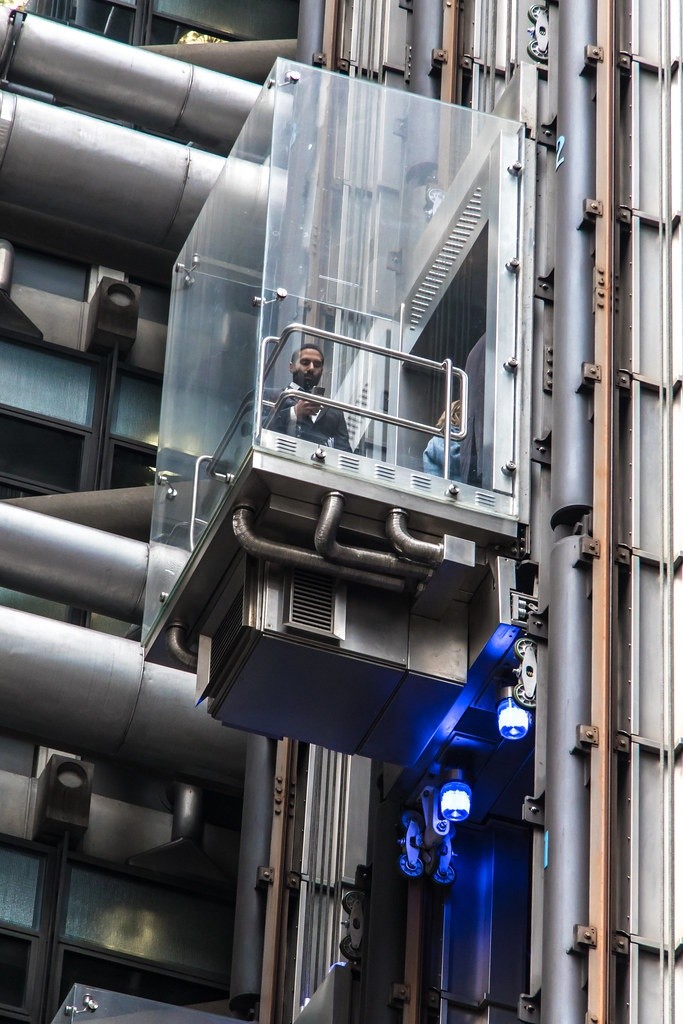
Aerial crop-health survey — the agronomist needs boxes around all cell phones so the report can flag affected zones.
[312,386,325,397]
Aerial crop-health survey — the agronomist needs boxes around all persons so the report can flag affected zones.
[423,400,462,483]
[262,343,353,454]
